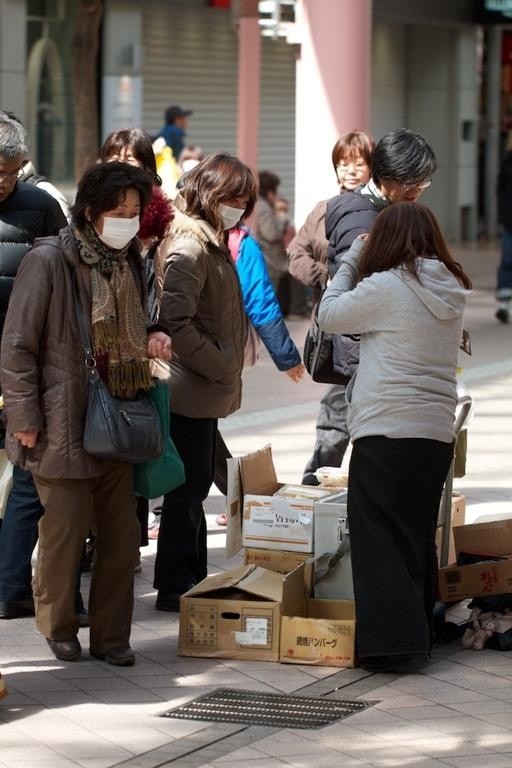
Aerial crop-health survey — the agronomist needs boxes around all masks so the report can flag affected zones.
[91,213,140,249]
[213,203,245,230]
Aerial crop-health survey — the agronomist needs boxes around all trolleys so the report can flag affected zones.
[432,395,512,651]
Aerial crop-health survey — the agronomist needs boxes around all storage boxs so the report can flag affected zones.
[223,444,315,554]
[242,548,315,600]
[314,489,356,600]
[433,444,510,654]
[180,563,281,664]
[279,561,358,666]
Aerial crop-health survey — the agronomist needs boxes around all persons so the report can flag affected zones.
[101,107,304,617]
[311,201,472,677]
[286,128,438,487]
[1,110,73,617]
[495,121,511,325]
[3,160,175,668]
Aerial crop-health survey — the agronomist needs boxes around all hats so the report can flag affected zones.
[165,106,193,120]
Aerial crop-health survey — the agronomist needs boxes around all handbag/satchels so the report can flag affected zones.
[83,370,164,465]
[129,378,187,500]
[303,299,347,384]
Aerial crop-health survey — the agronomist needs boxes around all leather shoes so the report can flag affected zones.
[89,641,135,666]
[156,580,195,611]
[78,609,90,627]
[47,635,82,661]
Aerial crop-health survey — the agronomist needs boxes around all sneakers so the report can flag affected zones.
[0,598,34,618]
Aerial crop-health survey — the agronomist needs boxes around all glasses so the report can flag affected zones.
[398,177,432,191]
[0,167,25,183]
[336,161,367,172]
